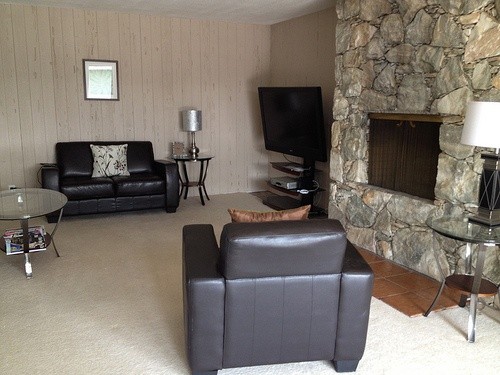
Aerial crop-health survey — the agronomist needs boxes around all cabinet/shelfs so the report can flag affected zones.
[265,161,324,217]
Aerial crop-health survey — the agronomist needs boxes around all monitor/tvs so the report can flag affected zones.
[257,86,328,171]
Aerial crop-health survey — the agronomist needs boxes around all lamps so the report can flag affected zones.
[182,109,203,155]
[461,101,500,228]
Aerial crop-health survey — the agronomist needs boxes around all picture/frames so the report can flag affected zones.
[83,59,120,101]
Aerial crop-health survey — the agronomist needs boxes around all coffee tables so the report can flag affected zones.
[0,185,66,279]
[172,153,214,206]
[426,203,500,344]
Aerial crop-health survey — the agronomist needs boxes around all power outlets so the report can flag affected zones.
[8,185,16,192]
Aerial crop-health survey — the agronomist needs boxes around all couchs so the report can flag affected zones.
[37,141,180,224]
[182,204,375,375]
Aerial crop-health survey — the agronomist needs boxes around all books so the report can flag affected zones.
[4,226,47,255]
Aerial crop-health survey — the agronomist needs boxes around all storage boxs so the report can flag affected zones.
[3,225,47,255]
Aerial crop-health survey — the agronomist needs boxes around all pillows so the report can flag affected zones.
[226,204,311,224]
[90,144,131,178]
